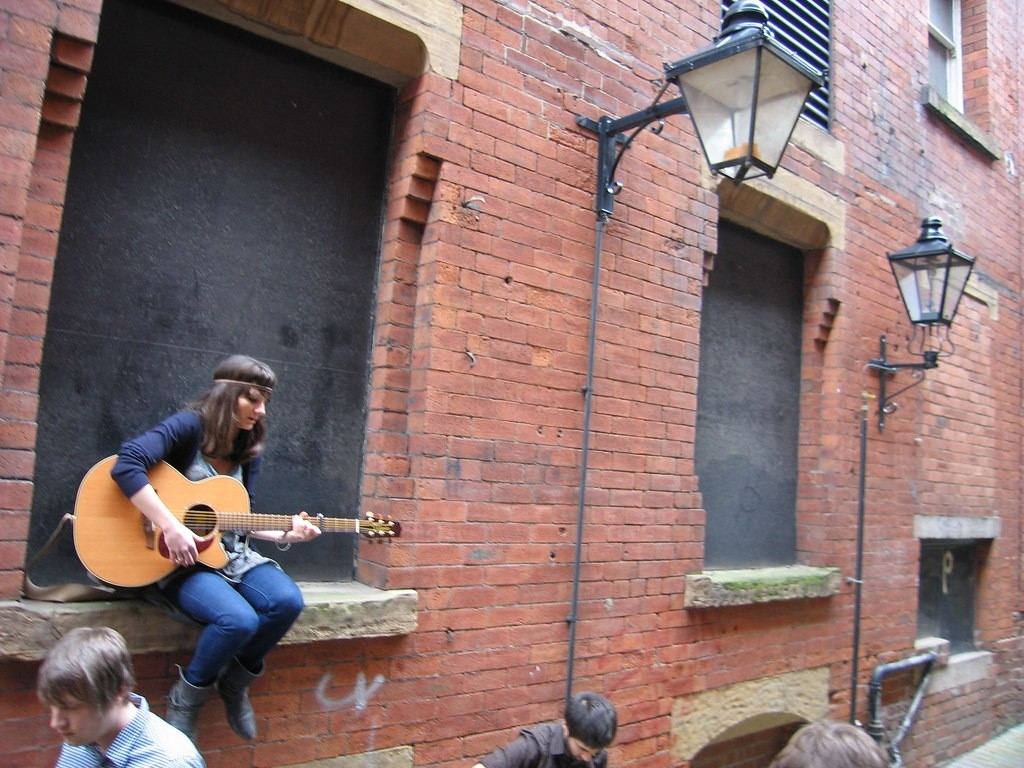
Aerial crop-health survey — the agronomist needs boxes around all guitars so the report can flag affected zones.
[72,452,402,595]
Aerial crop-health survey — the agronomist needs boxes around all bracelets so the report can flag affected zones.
[274,532,292,552]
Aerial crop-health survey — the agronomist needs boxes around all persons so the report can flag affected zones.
[110,354,323,745]
[471,691,618,768]
[34,625,208,768]
[767,718,894,768]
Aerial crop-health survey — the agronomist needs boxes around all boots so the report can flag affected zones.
[212,653,268,741]
[166,663,212,743]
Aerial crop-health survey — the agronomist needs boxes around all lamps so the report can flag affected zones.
[885,215,977,369]
[662,0,829,186]
